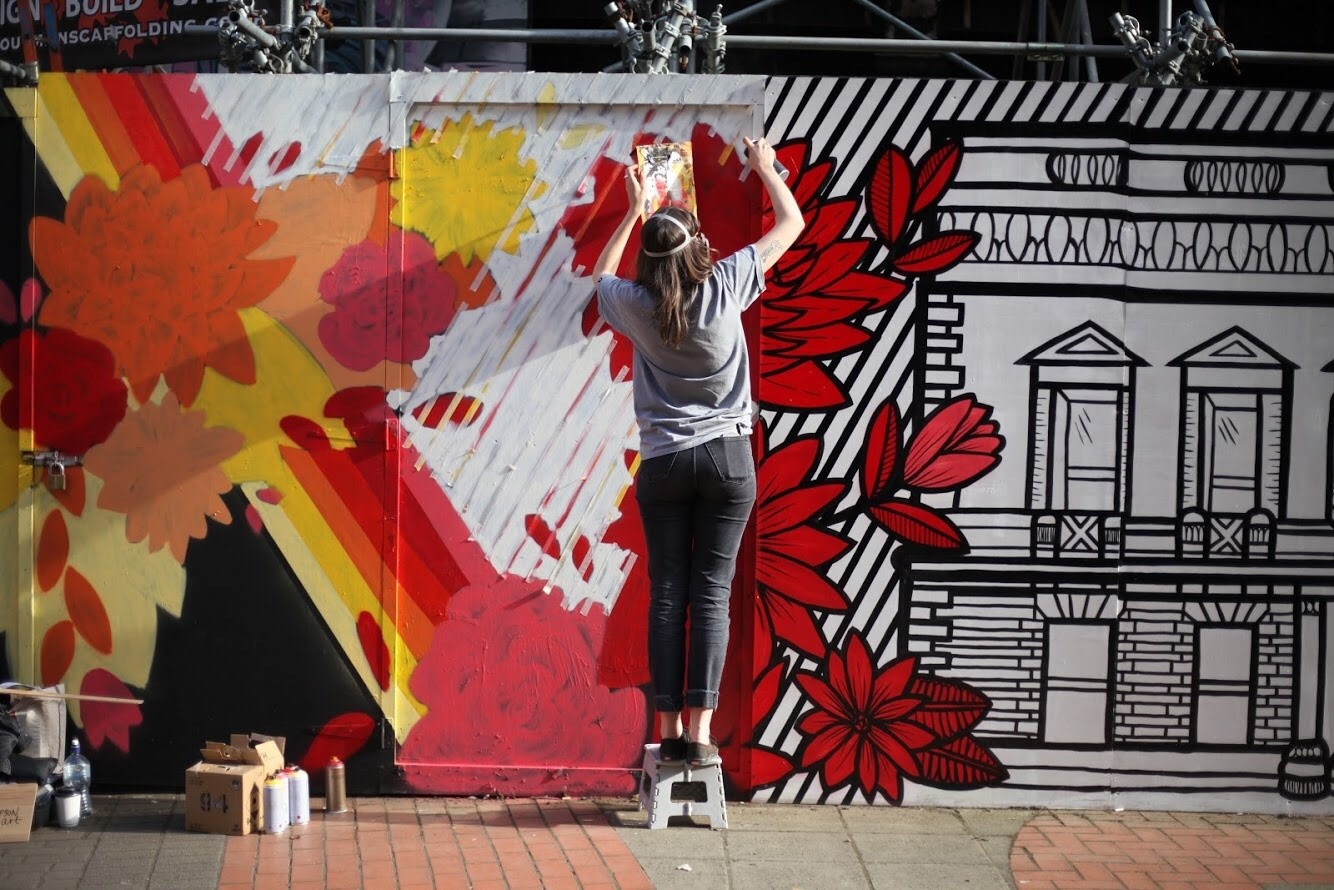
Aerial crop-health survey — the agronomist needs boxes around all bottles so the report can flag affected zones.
[325,756,347,814]
[743,140,790,182]
[274,761,310,826]
[63,739,93,819]
[264,776,286,835]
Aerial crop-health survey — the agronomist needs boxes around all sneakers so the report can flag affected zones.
[687,742,722,767]
[660,727,688,761]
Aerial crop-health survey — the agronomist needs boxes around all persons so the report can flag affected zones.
[593,136,805,765]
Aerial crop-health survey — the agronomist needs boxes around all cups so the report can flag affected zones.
[56,787,81,828]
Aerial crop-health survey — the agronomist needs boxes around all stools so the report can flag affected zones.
[637,743,729,831]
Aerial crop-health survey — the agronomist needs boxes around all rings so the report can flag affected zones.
[623,174,628,178]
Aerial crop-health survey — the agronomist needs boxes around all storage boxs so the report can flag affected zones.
[185,734,286,835]
[0,781,38,844]
